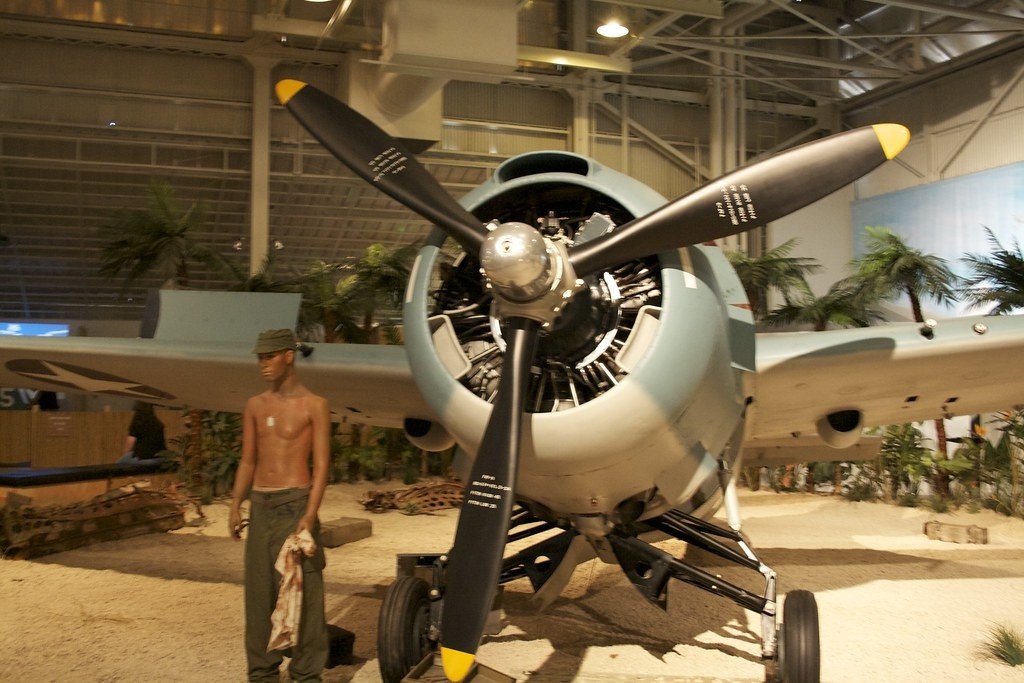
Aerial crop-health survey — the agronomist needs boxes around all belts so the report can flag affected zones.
[249,489,311,509]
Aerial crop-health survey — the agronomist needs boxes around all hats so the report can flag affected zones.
[252,328,297,355]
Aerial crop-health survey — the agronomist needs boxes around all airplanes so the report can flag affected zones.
[0,71,1024,683]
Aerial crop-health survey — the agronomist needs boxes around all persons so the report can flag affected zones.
[117,401,164,464]
[228,328,331,683]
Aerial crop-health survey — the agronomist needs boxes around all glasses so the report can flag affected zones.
[257,351,285,359]
